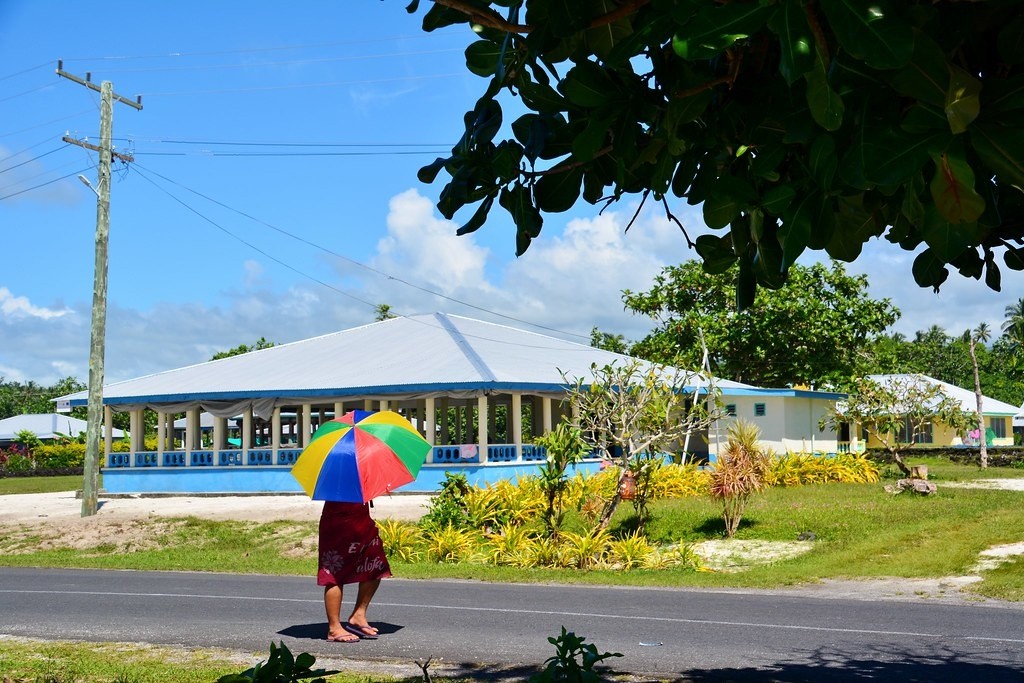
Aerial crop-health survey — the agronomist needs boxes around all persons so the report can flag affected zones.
[317,502,393,643]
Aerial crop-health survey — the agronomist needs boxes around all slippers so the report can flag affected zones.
[326,634,359,643]
[346,625,378,638]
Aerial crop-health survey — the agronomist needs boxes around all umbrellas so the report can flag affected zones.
[290,410,432,504]
